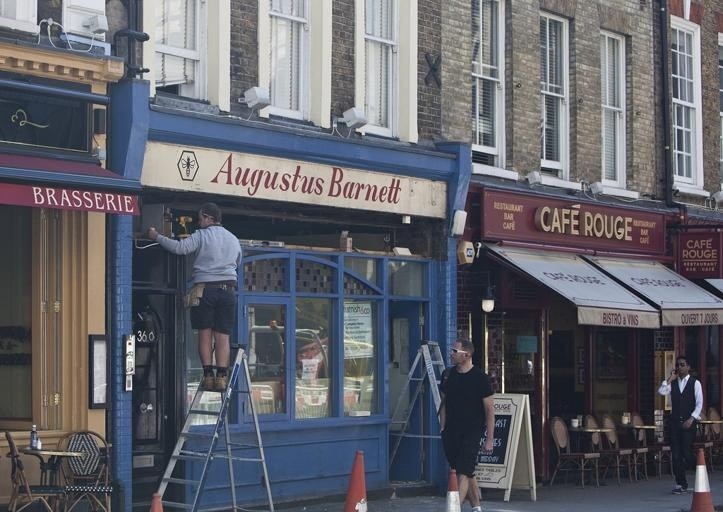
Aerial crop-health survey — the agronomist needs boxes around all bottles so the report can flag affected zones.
[29,425,41,451]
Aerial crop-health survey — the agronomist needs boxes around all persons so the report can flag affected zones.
[146,202,243,391]
[657,356,704,494]
[437,337,496,511]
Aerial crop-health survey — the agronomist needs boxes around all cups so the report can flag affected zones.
[621,416,628,424]
[570,419,578,429]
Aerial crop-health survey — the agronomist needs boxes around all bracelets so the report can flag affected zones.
[691,416,695,422]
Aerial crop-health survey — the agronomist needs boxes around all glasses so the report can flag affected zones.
[449,347,468,355]
[676,363,686,368]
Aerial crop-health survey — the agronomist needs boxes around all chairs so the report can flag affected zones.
[3,430,114,512]
[548,407,722,490]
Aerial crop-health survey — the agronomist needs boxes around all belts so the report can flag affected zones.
[205,284,226,288]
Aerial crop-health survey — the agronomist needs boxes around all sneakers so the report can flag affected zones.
[672,487,688,495]
[215,377,227,392]
[202,376,215,391]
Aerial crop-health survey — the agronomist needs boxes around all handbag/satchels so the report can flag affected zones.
[678,415,697,432]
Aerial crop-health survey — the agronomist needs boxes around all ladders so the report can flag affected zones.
[149,342,274,512]
[389,340,482,501]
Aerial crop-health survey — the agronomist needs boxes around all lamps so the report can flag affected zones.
[238,86,271,111]
[336,106,367,129]
[588,181,603,194]
[524,171,541,185]
[468,270,496,313]
[711,191,722,203]
[94,147,107,161]
[82,15,108,34]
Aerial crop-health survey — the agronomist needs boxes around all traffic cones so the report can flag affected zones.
[149,492,164,512]
[445,470,462,512]
[342,450,369,512]
[689,448,715,512]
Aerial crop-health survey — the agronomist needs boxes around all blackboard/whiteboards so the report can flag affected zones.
[473,393,525,490]
